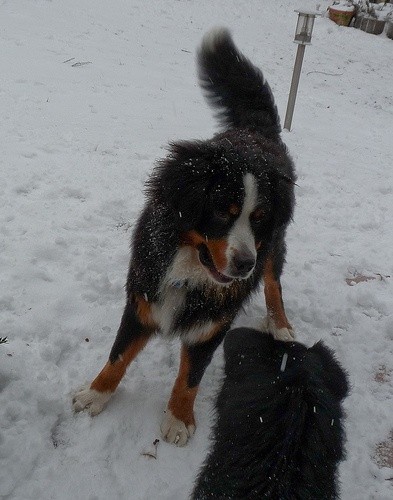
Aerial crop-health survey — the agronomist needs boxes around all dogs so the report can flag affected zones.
[187,326,352,500]
[72,27,298,448]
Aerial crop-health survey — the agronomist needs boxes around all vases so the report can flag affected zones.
[328,4,356,28]
[360,12,387,35]
